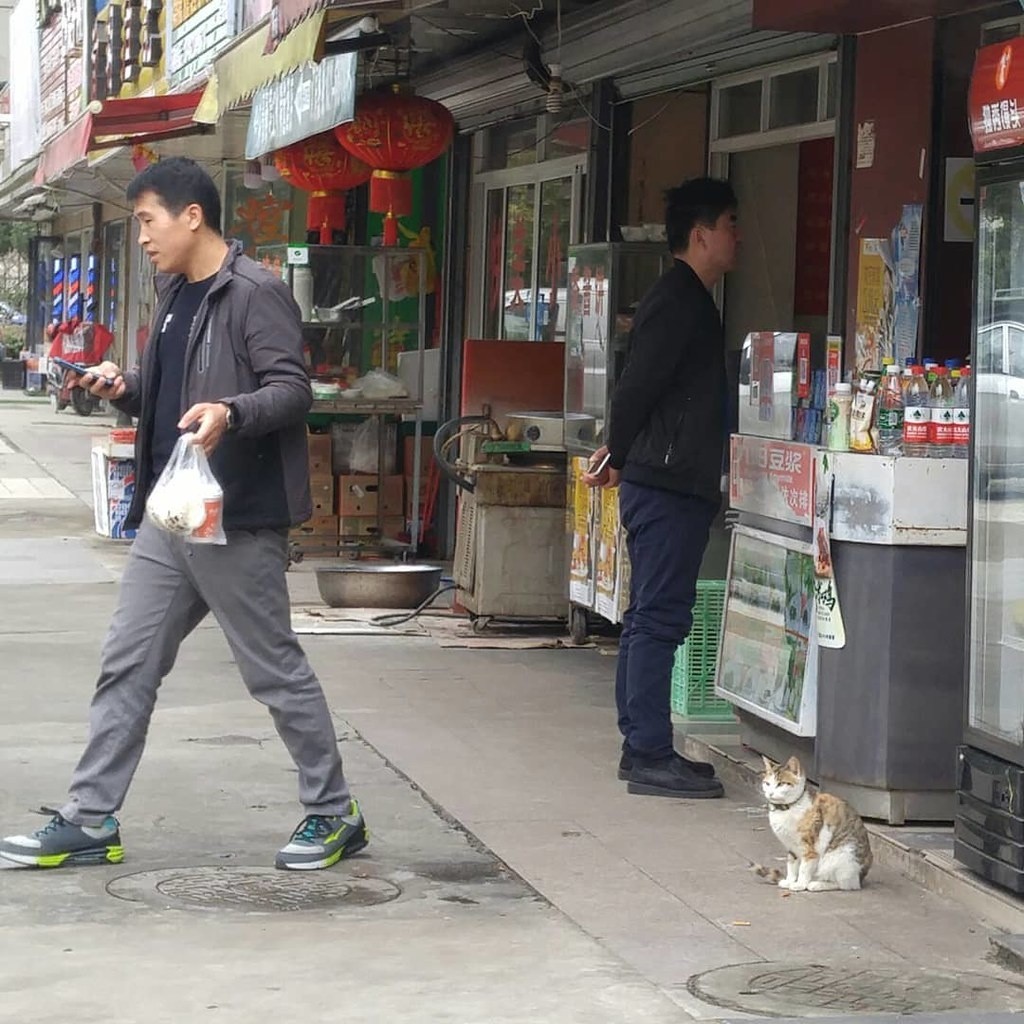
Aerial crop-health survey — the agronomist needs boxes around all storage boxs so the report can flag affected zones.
[288,424,435,558]
[671,580,735,722]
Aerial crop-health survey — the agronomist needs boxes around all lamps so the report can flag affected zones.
[261,152,280,181]
[244,160,262,189]
[12,193,65,222]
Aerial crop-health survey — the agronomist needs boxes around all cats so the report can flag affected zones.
[744,752,875,893]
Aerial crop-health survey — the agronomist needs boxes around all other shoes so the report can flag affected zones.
[618,751,716,780]
[628,760,725,798]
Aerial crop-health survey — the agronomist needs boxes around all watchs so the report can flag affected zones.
[226,405,234,429]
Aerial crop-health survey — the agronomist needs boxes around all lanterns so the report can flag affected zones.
[334,83,455,248]
[275,130,371,244]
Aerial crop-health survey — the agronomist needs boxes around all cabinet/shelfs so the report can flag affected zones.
[255,243,426,407]
[563,241,669,454]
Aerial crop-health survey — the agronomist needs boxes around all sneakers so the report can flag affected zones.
[0,806,123,869]
[275,798,372,870]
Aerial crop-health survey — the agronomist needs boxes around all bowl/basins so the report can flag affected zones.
[314,308,358,323]
[620,223,667,243]
[314,563,444,609]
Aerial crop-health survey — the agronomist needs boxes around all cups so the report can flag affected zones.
[184,495,222,543]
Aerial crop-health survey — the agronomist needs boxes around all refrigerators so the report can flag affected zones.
[950,43,1024,902]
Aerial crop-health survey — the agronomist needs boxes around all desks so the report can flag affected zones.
[286,398,421,562]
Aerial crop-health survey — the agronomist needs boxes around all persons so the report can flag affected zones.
[583,176,742,799]
[0,156,368,868]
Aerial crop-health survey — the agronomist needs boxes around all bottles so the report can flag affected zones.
[875,356,971,459]
[261,253,313,321]
[826,383,852,453]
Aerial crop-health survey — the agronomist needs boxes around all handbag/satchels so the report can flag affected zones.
[146,437,229,546]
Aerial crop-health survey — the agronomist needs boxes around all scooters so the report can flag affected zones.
[48,319,103,417]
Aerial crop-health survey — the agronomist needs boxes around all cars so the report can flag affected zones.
[737,330,800,398]
[978,320,1024,499]
[504,288,566,343]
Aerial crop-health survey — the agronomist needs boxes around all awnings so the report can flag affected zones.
[0,152,131,221]
[40,91,212,213]
[260,0,334,55]
[192,0,411,124]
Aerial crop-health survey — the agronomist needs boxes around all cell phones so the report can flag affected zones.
[588,450,612,475]
[54,357,114,386]
